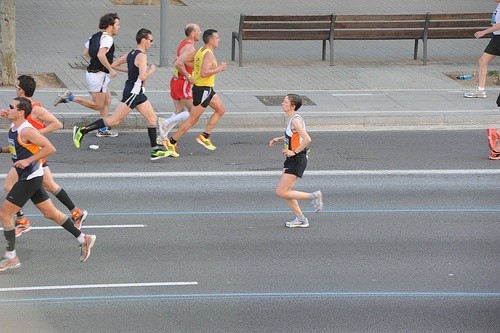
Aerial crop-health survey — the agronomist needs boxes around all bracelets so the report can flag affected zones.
[293,150,297,155]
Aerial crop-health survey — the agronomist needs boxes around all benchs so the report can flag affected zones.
[321,11,431,66]
[413,10,494,67]
[231,12,337,67]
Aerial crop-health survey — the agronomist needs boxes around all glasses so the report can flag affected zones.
[9,105,19,109]
[147,38,153,42]
[15,85,24,92]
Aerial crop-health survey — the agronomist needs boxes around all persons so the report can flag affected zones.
[53,12,124,137]
[0,74,90,238]
[464,0,500,99]
[155,24,208,145]
[487,91,500,160]
[0,97,97,272]
[269,93,323,228]
[72,28,173,161]
[161,28,228,158]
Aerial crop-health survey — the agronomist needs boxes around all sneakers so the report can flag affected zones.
[195,134,216,151]
[0,256,22,272]
[149,149,172,160]
[311,190,322,213]
[96,127,118,137]
[164,138,180,157]
[158,117,166,139]
[155,137,163,145]
[78,235,96,262]
[14,218,32,238]
[464,86,487,97]
[487,128,499,151]
[53,89,73,106]
[71,208,88,231]
[73,125,84,149]
[489,150,500,160]
[285,217,309,228]
